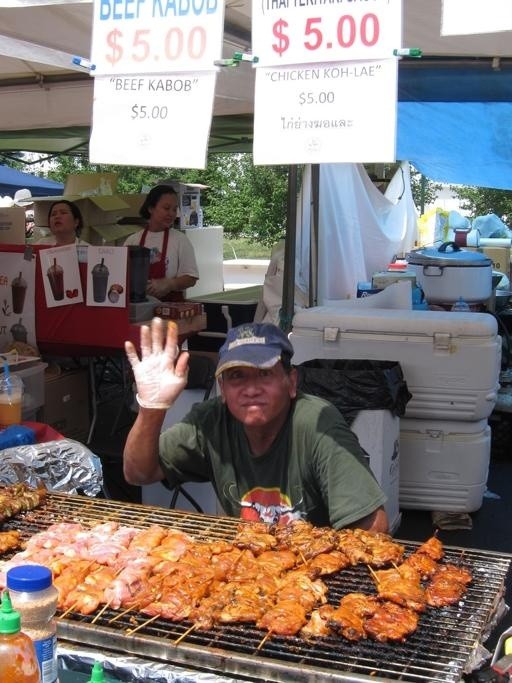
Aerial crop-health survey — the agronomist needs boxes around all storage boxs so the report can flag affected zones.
[18,170,210,246]
[35,363,91,445]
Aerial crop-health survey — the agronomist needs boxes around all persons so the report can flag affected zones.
[117,310,392,535]
[118,183,197,302]
[43,200,94,263]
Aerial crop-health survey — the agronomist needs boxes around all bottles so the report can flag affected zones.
[450,295,470,311]
[0,566,64,683]
[84,658,119,683]
[411,280,429,310]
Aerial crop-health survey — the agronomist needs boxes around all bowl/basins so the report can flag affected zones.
[490,272,512,312]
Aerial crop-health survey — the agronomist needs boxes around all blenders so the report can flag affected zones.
[125,244,162,321]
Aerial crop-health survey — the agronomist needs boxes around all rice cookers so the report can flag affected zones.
[404,240,494,307]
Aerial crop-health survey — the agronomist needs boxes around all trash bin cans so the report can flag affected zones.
[296,359,413,538]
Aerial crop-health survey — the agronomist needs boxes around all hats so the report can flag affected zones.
[215,322,294,379]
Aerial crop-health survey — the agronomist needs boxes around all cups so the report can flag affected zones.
[9,324,28,343]
[10,277,28,314]
[0,372,25,428]
[47,265,65,300]
[92,263,109,303]
